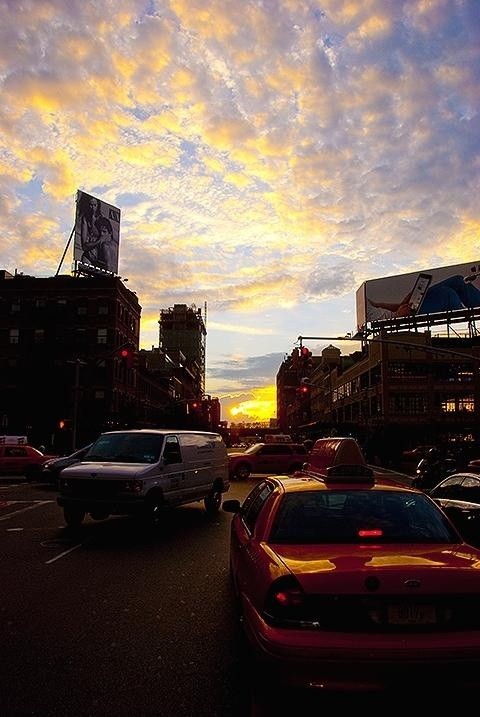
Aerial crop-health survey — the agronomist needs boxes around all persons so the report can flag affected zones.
[367,274,480,321]
[78,194,111,266]
[416,449,439,480]
[96,217,118,273]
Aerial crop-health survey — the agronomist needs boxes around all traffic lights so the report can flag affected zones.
[289,349,298,370]
[302,346,311,359]
[300,377,311,393]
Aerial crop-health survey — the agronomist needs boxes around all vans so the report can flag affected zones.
[58,430,231,538]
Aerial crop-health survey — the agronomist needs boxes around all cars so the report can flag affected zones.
[0,436,95,486]
